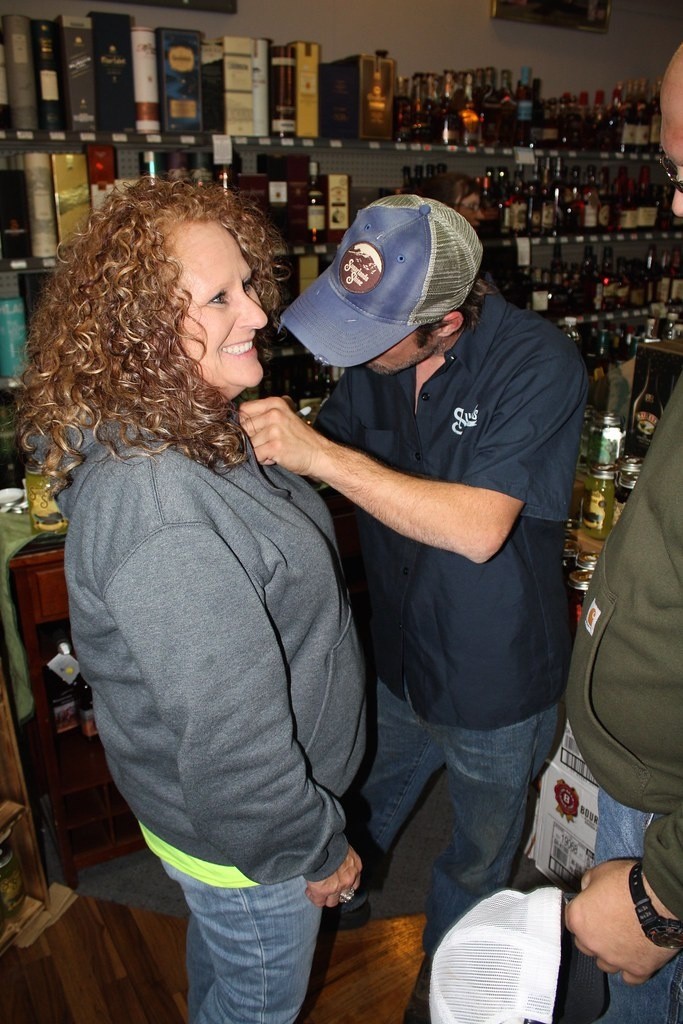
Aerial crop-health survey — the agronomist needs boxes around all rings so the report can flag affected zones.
[339,888,355,904]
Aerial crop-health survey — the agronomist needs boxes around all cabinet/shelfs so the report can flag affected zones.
[0,666,50,960]
[0,129,683,476]
[6,527,153,892]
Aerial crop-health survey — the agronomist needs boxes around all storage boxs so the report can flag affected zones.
[520,714,604,899]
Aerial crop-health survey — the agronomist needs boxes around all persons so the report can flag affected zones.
[13,174,368,1024]
[240,194,590,1024]
[566,42,683,1024]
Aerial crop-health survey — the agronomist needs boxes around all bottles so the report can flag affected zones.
[391,66,683,384]
[305,162,326,244]
[262,357,336,427]
[630,358,663,457]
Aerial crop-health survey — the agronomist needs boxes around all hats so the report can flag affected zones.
[279,194,483,368]
[430,887,606,1024]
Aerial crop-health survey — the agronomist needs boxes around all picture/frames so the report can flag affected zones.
[491,0,611,35]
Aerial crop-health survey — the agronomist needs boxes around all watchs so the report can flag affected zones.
[628,862,683,949]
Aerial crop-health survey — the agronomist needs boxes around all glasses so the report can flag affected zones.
[661,153,683,193]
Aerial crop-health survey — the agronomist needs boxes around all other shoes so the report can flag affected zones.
[321,896,371,929]
[404,954,433,1024]
